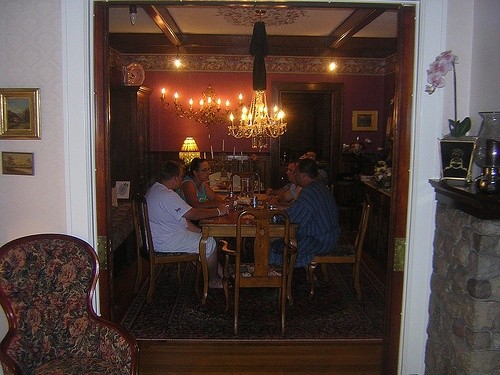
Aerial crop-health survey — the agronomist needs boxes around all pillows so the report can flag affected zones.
[111,187,118,207]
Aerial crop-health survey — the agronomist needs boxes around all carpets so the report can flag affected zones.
[120,252,387,342]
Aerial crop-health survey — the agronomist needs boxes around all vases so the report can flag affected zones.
[437,133,478,183]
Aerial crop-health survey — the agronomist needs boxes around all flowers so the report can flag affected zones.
[425,50,471,136]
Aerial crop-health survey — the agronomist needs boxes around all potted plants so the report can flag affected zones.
[333,146,377,203]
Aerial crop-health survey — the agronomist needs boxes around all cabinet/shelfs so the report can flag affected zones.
[111,85,152,191]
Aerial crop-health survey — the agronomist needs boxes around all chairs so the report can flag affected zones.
[132,193,201,304]
[219,209,297,337]
[303,201,370,302]
[0,234,139,375]
[231,172,260,194]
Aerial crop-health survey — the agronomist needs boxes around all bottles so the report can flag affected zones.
[473,111,500,192]
[353,136,364,151]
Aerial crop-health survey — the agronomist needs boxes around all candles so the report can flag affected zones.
[233,146,236,157]
[210,146,214,159]
[222,138,225,151]
[241,151,244,164]
[204,152,206,159]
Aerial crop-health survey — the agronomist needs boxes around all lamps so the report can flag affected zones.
[160,8,289,165]
[129,4,137,25]
[326,47,337,72]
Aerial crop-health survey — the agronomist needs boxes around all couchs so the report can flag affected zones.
[111,172,136,250]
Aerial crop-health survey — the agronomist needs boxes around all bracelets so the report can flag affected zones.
[216,208,221,216]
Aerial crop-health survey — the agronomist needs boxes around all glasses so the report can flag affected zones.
[305,155,316,159]
[293,171,304,175]
[195,167,210,172]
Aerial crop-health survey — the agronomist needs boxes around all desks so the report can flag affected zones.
[338,205,359,234]
[112,200,134,251]
[424,179,500,375]
[198,194,297,308]
[360,176,391,261]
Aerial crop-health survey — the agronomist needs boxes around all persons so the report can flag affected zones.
[268,158,338,268]
[141,162,236,289]
[266,151,329,205]
[175,157,234,225]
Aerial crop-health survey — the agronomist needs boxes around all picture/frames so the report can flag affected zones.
[1,151,33,176]
[0,87,41,140]
[351,111,378,131]
